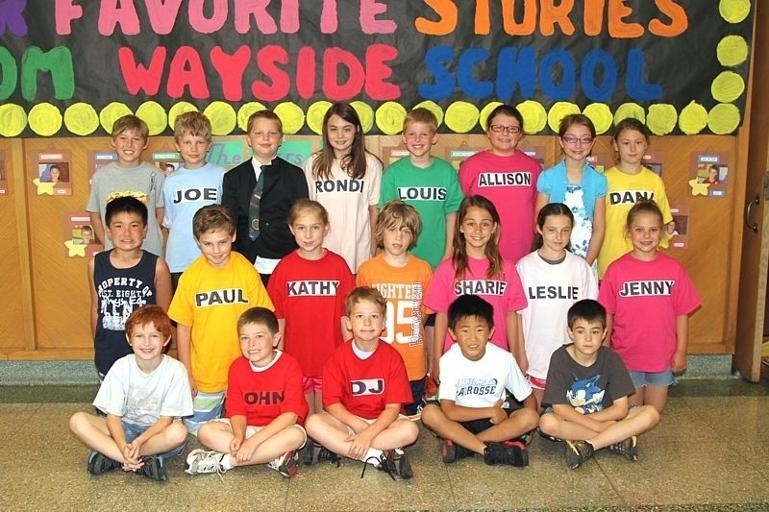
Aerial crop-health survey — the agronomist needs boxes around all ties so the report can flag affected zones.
[248,165,268,241]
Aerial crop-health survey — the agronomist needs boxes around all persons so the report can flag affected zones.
[597,117,674,290]
[266,199,355,415]
[702,164,719,184]
[533,114,609,287]
[305,100,383,275]
[514,203,600,416]
[163,164,175,175]
[375,107,466,270]
[458,104,544,265]
[160,111,227,326]
[46,164,61,181]
[597,199,704,414]
[354,199,437,421]
[166,203,276,438]
[303,286,419,481]
[536,299,661,470]
[221,111,309,288]
[87,196,173,417]
[183,307,308,479]
[83,114,166,259]
[69,305,195,481]
[79,225,96,244]
[422,194,529,405]
[420,294,540,467]
[664,220,679,235]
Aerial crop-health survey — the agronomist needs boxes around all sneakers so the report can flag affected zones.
[267,450,300,477]
[442,439,474,463]
[606,436,638,461]
[376,448,413,479]
[137,455,168,480]
[184,449,226,473]
[304,441,342,468]
[565,439,594,470]
[88,450,122,474]
[484,441,528,466]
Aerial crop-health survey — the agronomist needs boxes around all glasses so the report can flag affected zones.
[563,136,593,143]
[490,125,520,133]
